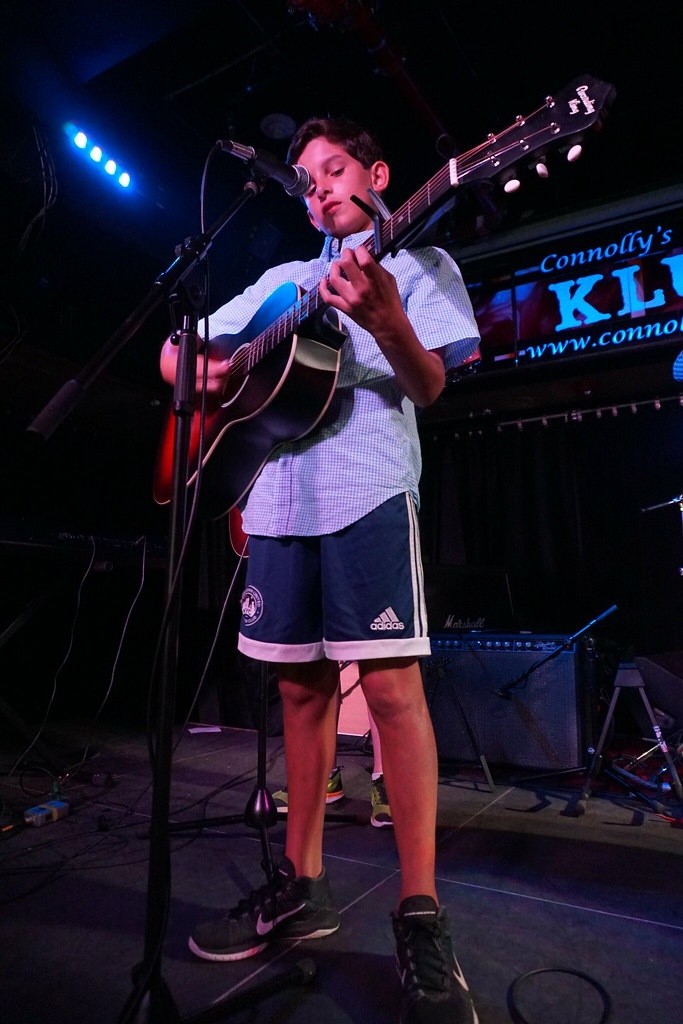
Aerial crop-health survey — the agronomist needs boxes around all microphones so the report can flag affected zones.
[491,689,513,700]
[215,139,311,198]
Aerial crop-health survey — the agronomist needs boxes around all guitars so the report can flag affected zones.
[228,340,484,561]
[147,72,620,527]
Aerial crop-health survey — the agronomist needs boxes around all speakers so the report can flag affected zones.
[334,660,374,748]
[418,630,600,776]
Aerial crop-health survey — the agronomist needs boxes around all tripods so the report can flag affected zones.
[166,660,372,878]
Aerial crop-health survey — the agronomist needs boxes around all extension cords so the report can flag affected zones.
[24,799,68,827]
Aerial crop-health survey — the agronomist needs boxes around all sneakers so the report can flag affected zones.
[188,856,340,961]
[389,895,480,1024]
[271,766,345,813]
[371,775,393,828]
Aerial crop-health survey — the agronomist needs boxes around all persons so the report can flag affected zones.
[269,702,395,829]
[156,117,480,1024]
